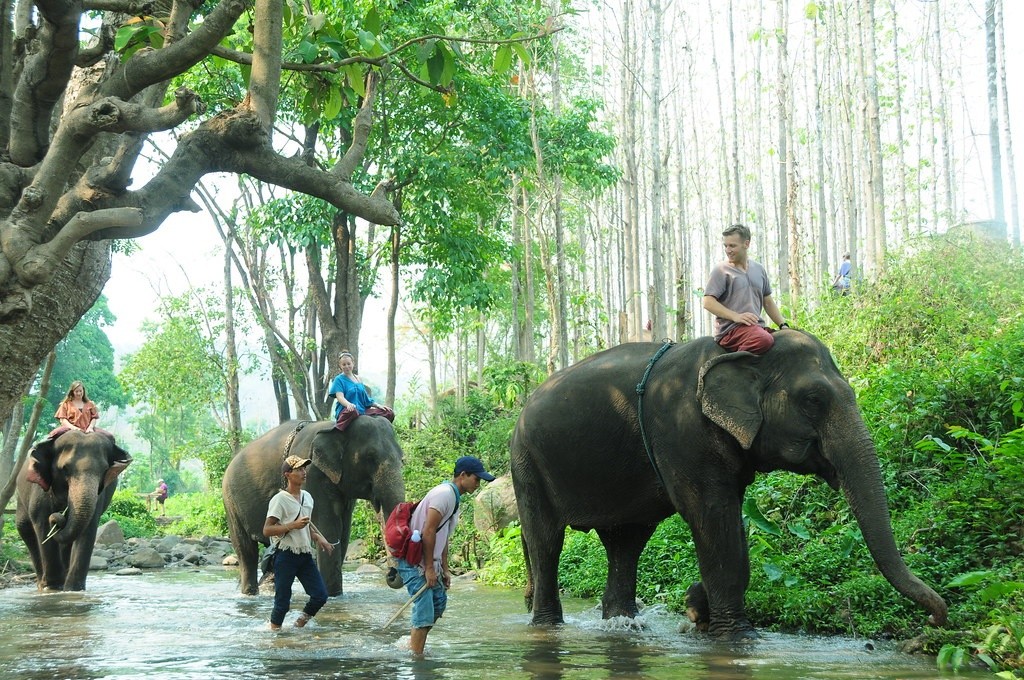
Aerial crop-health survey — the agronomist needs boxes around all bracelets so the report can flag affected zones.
[779,323,789,330]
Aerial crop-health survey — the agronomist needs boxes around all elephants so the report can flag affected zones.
[15,428,134,591]
[509,328,947,647]
[222,415,405,597]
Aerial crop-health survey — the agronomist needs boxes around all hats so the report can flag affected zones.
[281,455,312,477]
[454,455,495,482]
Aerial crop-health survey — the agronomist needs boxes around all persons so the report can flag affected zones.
[262,455,334,631]
[702,224,790,354]
[329,350,396,431]
[389,457,497,653]
[50,381,115,443]
[151,479,168,516]
[834,253,861,290]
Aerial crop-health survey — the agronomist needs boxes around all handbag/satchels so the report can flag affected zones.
[261,544,278,576]
[385,501,415,559]
